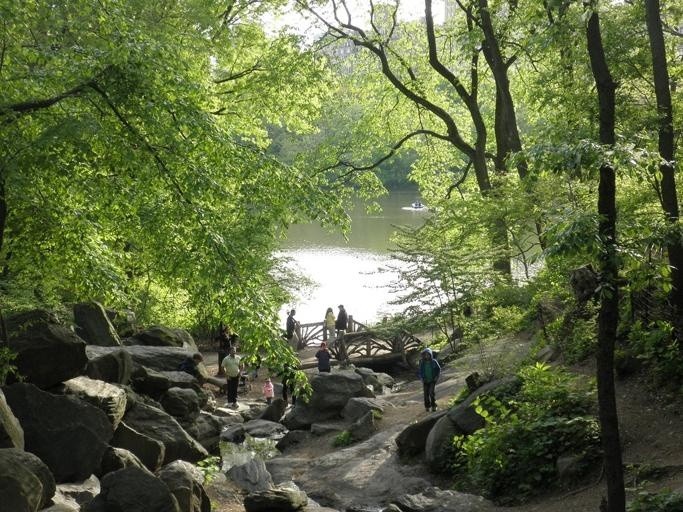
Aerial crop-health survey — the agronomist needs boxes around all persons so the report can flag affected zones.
[325,307,335,341]
[275,361,297,406]
[179,353,203,377]
[417,348,440,413]
[222,346,244,408]
[263,377,275,407]
[314,342,333,372]
[216,322,234,375]
[335,304,347,336]
[287,310,300,351]
[252,353,262,381]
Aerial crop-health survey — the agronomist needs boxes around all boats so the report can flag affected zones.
[412,203,424,209]
